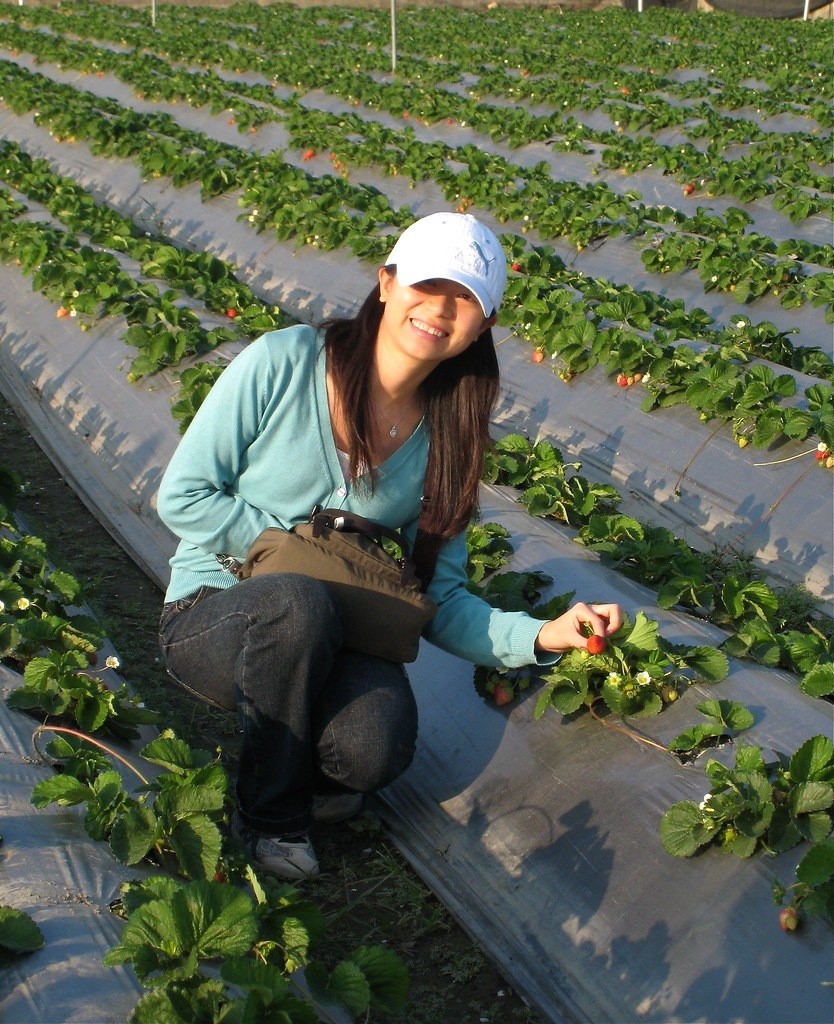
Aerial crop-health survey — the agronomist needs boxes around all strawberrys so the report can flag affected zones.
[5,38,834,930]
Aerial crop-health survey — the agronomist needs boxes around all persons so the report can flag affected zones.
[157,213,624,877]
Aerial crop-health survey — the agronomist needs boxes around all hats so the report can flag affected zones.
[385,211,507,318]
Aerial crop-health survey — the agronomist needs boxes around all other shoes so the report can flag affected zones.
[254,831,318,879]
[312,793,350,820]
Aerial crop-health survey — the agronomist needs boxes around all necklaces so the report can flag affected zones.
[369,399,416,438]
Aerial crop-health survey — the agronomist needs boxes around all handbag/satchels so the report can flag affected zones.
[235,503,438,665]
